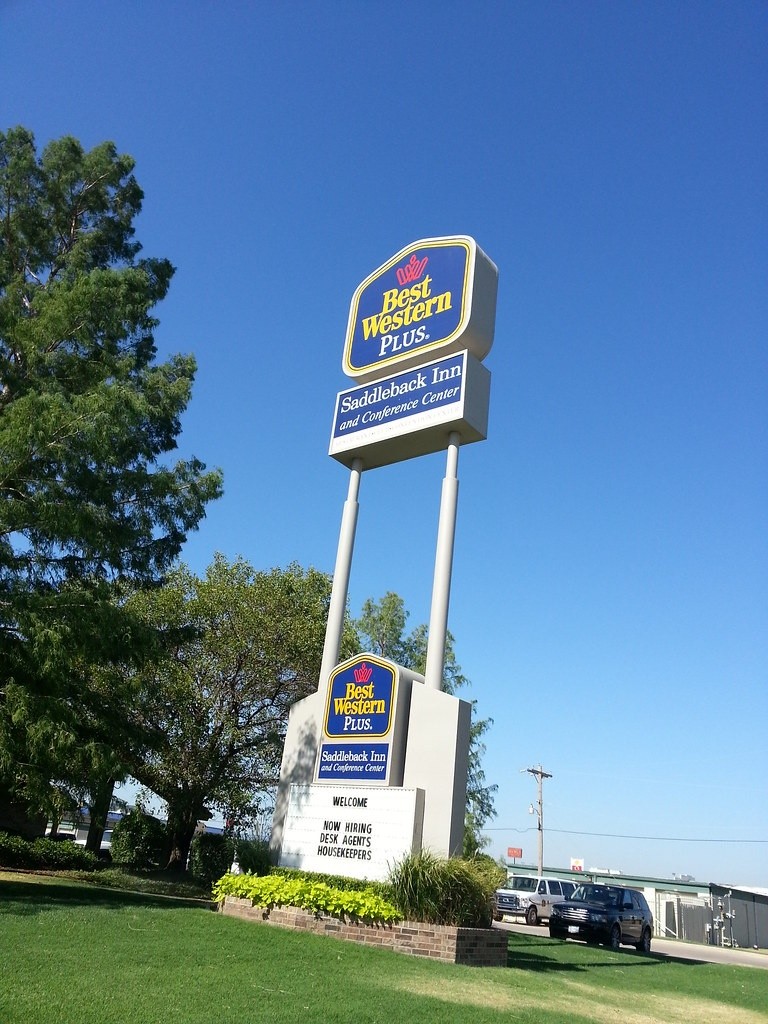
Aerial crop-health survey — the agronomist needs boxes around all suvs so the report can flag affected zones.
[550,884,654,951]
[492,875,580,925]
[73,829,116,863]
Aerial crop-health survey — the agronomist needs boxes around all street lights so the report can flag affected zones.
[529,804,544,877]
